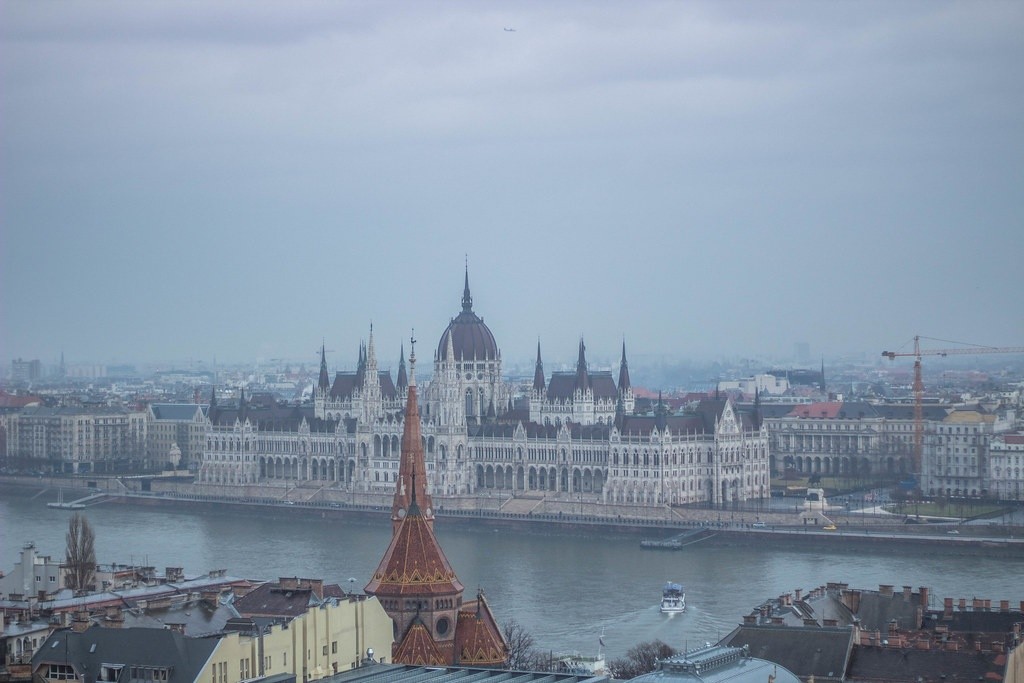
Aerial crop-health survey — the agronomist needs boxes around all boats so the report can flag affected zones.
[641,539,683,550]
[47,489,86,509]
[660,581,685,613]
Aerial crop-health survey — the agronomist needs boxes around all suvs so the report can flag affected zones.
[754,522,767,527]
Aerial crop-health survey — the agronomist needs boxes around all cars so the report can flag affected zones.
[824,525,837,530]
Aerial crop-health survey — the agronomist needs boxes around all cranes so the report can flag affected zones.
[883,336,1024,496]
